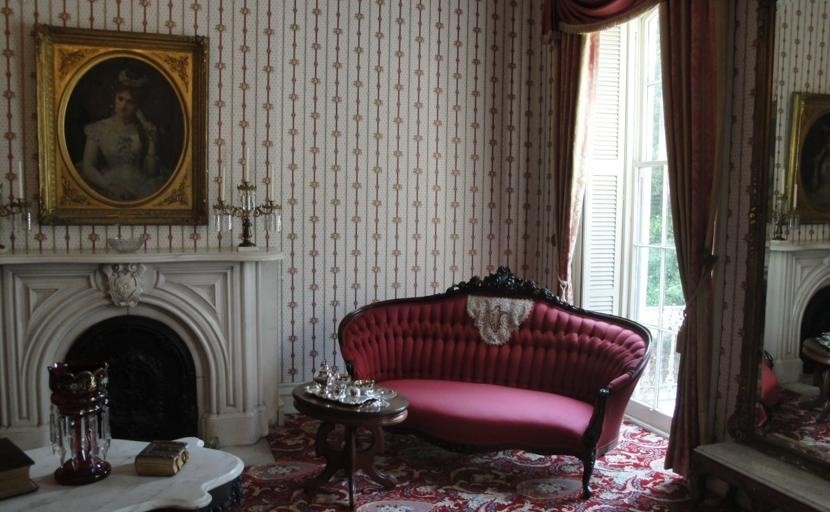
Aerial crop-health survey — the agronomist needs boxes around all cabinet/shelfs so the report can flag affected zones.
[691,438,830,512]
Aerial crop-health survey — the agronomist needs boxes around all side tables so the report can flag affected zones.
[800,336,830,422]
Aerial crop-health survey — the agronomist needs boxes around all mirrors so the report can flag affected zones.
[729,0,830,479]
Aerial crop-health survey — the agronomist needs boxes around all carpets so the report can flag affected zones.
[146,411,693,509]
[759,388,830,462]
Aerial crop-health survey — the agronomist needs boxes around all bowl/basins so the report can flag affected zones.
[106,238,144,253]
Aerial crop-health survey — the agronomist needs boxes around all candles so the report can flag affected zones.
[17,161,23,195]
[781,168,785,196]
[221,166,226,200]
[270,165,274,200]
[793,182,797,209]
[246,146,250,181]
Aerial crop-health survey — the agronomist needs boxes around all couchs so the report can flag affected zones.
[339,264,654,498]
[756,346,777,424]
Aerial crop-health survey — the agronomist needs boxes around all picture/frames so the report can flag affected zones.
[783,90,830,223]
[31,21,211,226]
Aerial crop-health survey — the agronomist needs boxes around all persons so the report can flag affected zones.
[83,70,176,201]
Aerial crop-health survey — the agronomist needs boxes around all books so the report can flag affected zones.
[135,440,189,477]
[0,438,39,500]
